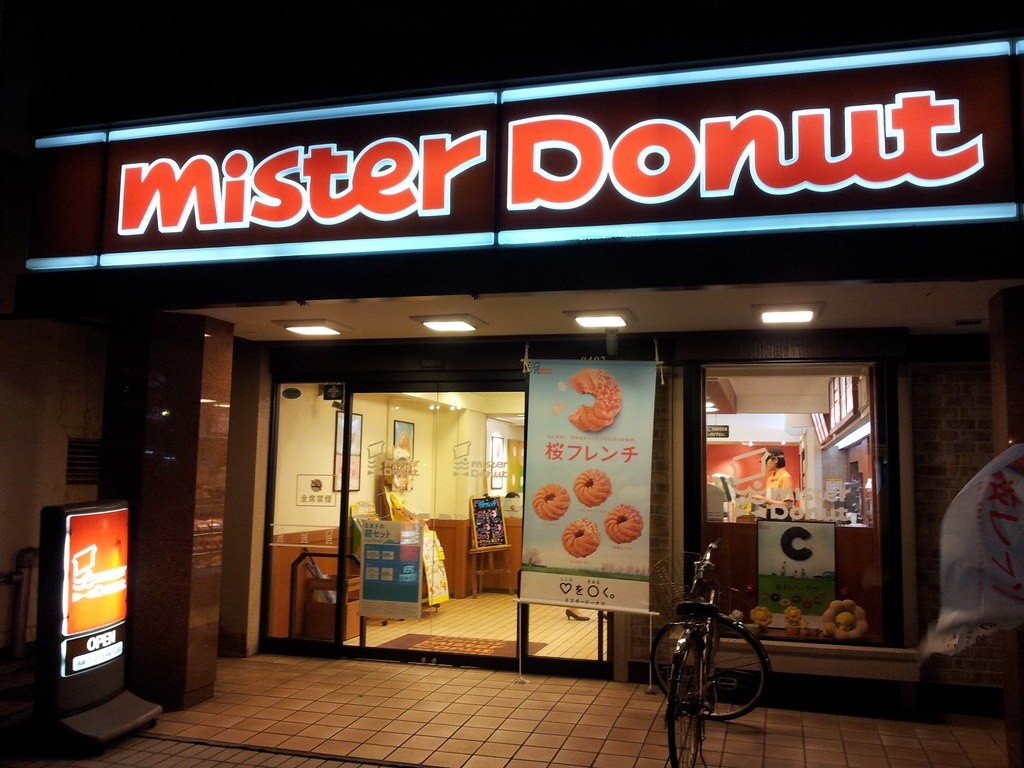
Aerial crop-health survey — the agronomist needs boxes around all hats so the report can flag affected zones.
[758,451,784,463]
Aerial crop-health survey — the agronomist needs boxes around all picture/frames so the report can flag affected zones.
[491,436,505,489]
[393,420,415,491]
[333,410,363,491]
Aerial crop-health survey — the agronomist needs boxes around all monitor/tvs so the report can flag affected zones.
[720,476,736,502]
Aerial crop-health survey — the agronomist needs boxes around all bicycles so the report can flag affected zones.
[645,542,774,768]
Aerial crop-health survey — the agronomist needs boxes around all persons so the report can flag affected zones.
[746,447,795,521]
[504,492,521,499]
[565,607,609,622]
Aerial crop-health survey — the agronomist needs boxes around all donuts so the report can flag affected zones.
[532,483,570,521]
[605,503,642,545]
[562,517,601,558]
[572,468,612,507]
[568,368,622,431]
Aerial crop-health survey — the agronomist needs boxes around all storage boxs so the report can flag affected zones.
[304,574,362,641]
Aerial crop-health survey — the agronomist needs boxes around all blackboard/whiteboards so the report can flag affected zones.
[469,496,511,554]
